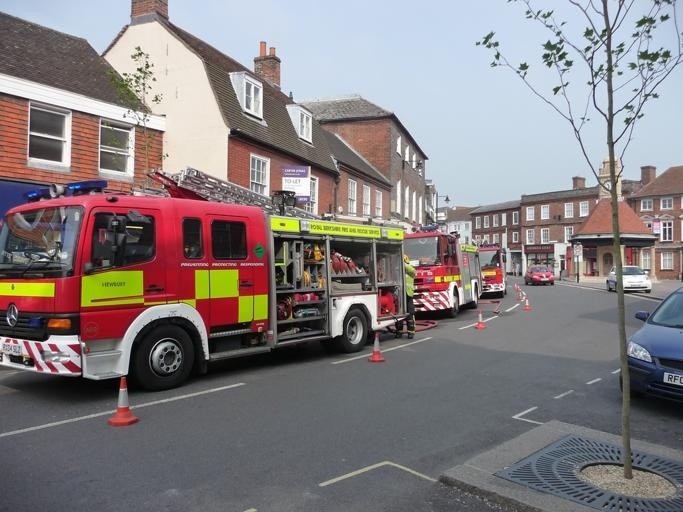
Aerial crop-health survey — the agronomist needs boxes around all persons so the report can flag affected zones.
[392,254,417,338]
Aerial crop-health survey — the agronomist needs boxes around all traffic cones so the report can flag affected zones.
[368,333,385,362]
[108,377,140,426]
[475,310,486,329]
[514,283,531,310]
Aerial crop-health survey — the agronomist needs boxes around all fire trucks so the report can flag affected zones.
[471,239,507,298]
[403,222,482,318]
[0,167,410,391]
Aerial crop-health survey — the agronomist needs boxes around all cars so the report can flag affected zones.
[620,287,683,406]
[606,266,652,293]
[525,265,554,286]
